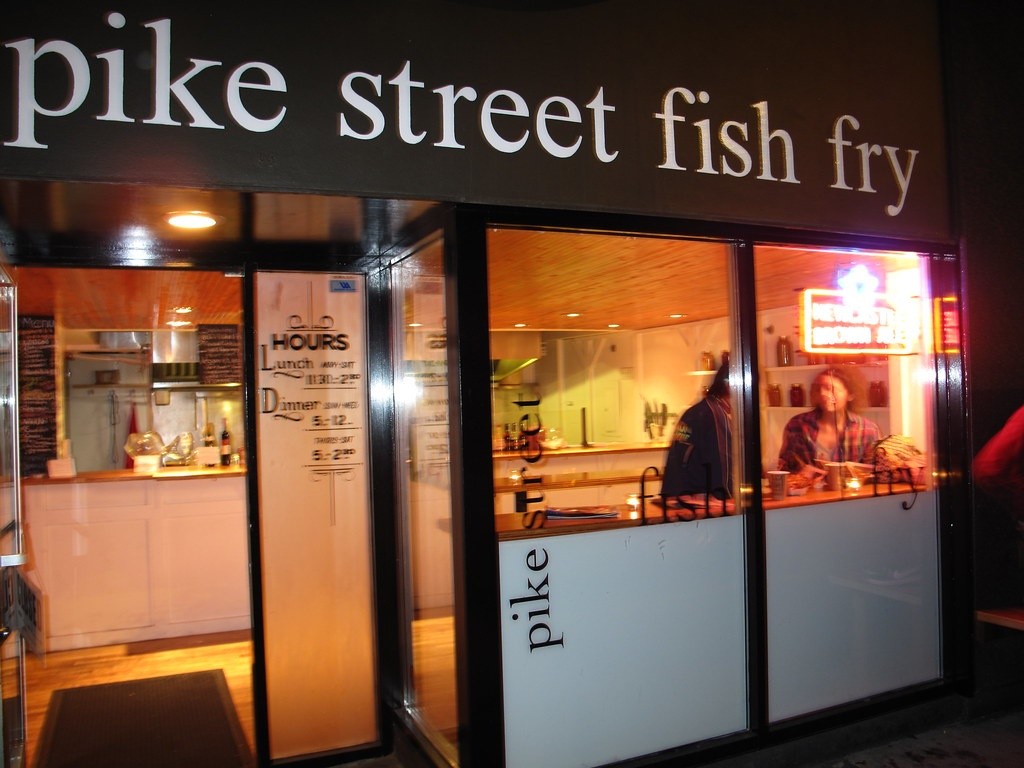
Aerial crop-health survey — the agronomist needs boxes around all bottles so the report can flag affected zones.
[768,383,780,407]
[205,423,215,467]
[870,380,884,407]
[721,350,728,366]
[219,418,231,466]
[790,383,804,407]
[777,335,791,367]
[493,423,527,451]
[702,350,716,371]
[702,385,710,400]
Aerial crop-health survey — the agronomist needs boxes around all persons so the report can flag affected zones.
[777,368,881,473]
[661,362,734,501]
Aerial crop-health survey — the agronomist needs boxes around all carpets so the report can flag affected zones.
[32,667,257,768]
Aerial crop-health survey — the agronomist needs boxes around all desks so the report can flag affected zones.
[494,473,919,541]
[494,464,668,509]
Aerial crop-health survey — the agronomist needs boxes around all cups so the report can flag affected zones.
[825,464,847,491]
[767,470,790,501]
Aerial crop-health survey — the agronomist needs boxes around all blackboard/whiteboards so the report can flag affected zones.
[16,315,57,477]
[196,323,240,385]
